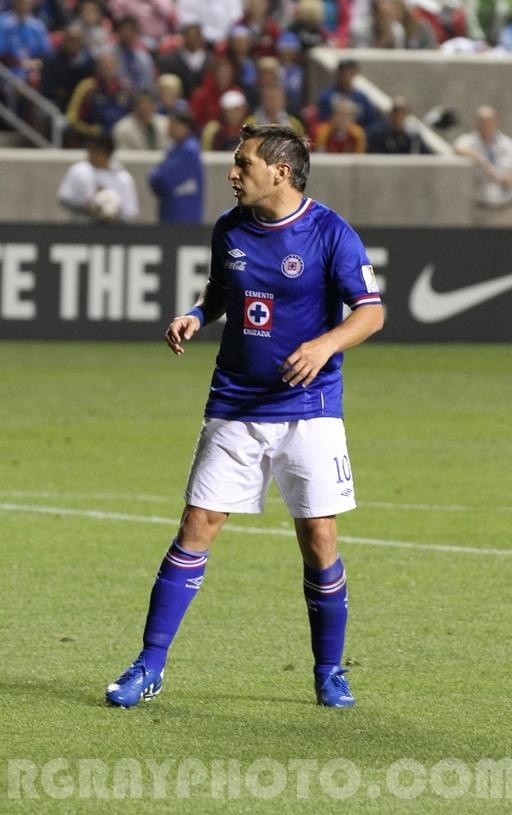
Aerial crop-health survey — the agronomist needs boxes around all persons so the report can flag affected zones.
[104,123,384,709]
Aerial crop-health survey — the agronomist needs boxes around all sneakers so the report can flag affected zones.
[313,664,356,707]
[105,657,165,707]
